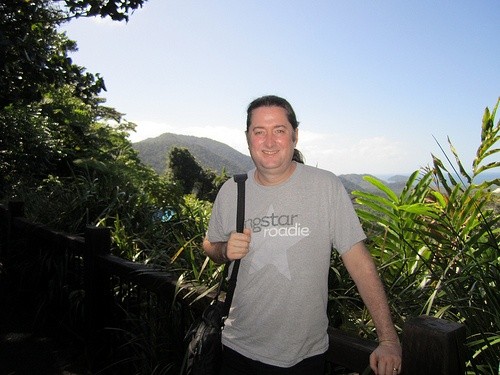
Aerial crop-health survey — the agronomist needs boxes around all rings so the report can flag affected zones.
[393,367,398,371]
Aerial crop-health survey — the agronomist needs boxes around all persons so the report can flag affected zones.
[202,96,403,375]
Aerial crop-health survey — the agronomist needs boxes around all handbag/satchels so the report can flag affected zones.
[180,300,224,375]
